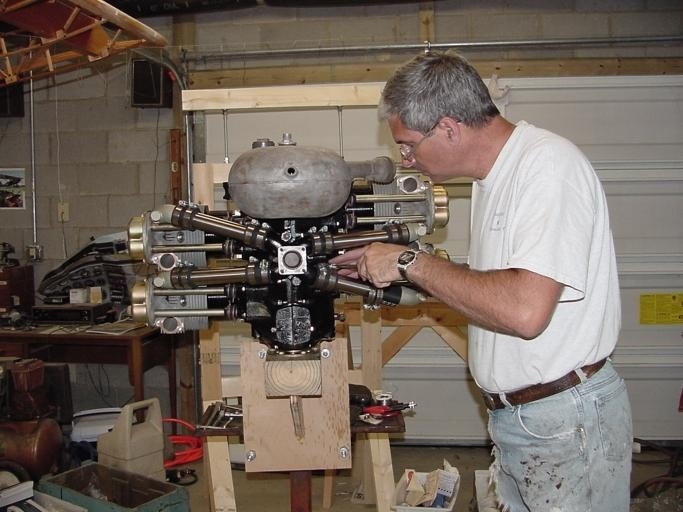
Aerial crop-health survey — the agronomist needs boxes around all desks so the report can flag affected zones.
[0,320,178,456]
[195,400,406,512]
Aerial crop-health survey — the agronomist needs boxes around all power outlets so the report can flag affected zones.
[29,248,36,260]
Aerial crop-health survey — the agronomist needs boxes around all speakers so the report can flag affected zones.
[0,85,24,117]
[128,58,173,108]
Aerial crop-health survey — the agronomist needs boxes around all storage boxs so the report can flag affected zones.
[474,469,502,512]
[38,461,188,512]
[390,470,461,512]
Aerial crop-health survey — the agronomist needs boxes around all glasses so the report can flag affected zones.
[400,117,462,163]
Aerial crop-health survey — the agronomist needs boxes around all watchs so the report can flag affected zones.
[396,248,422,282]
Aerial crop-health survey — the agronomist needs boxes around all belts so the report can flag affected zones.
[481,357,607,409]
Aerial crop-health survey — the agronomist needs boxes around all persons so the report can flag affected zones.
[329,47,636,511]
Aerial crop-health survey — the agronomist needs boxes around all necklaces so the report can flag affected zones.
[477,124,517,180]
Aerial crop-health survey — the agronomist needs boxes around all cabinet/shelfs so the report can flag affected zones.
[0,266,35,359]
